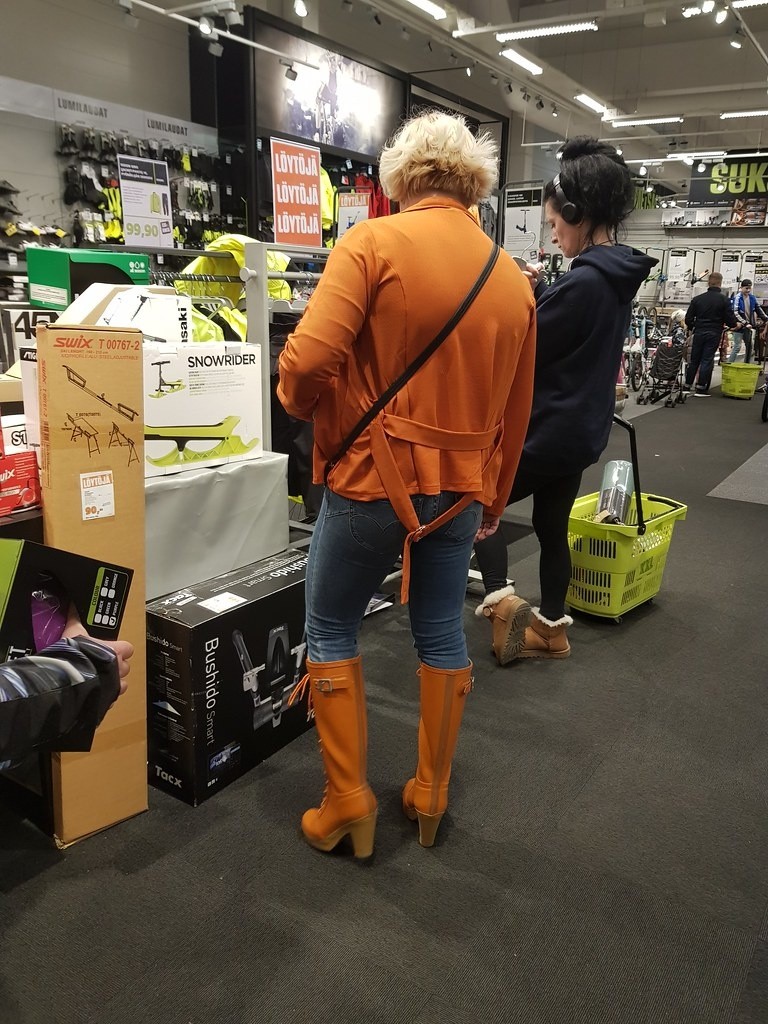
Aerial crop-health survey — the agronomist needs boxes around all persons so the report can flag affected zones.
[728,278,768,363]
[275,110,539,859]
[682,272,749,397]
[667,308,689,389]
[0,604,135,764]
[682,332,702,389]
[753,320,768,394]
[478,131,660,669]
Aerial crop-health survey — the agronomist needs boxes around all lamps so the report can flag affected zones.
[110,0,768,208]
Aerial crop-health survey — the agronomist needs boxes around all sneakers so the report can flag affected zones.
[694,389,711,397]
[682,386,690,394]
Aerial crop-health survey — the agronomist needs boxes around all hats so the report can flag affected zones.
[741,279,752,287]
[671,309,686,323]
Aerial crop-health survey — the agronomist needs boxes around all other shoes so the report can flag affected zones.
[754,383,767,394]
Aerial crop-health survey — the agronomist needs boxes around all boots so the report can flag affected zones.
[475,586,573,664]
[404,661,474,847]
[301,656,379,858]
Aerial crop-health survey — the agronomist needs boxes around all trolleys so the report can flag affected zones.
[719,324,763,401]
[559,413,687,625]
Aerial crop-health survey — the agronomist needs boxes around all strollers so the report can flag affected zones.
[636,329,691,408]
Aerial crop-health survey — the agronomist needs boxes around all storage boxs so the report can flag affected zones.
[1,247,339,847]
